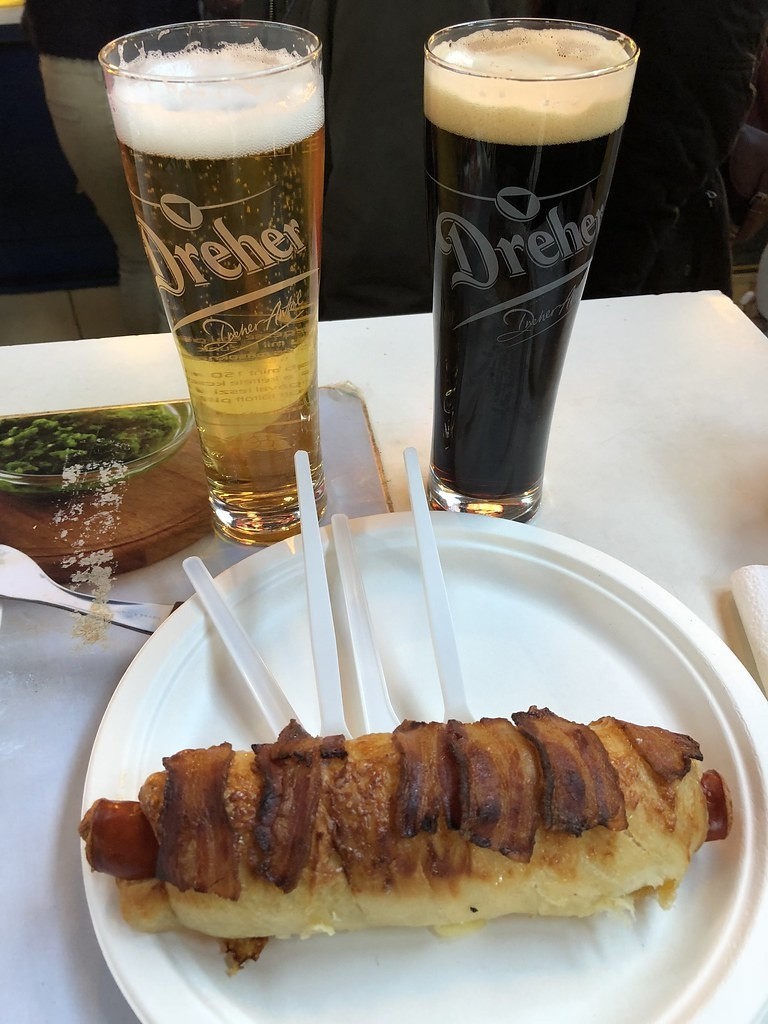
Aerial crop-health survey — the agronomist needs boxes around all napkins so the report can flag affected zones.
[732,565,767,695]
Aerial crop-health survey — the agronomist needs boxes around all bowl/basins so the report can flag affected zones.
[2,398,194,498]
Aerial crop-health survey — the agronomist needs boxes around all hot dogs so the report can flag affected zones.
[78,705,734,954]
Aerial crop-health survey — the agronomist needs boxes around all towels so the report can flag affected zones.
[731,563,768,699]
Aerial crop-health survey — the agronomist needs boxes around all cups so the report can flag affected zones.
[424,15,641,527]
[96,19,327,545]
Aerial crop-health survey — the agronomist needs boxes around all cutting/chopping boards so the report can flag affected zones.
[1,422,215,588]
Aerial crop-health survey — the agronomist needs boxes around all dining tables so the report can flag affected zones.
[0,289,767,1024]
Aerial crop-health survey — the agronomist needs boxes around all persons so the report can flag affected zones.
[199,0,538,318]
[24,1,200,335]
[530,0,768,297]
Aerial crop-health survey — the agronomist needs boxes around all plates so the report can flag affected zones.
[80,513,766,1023]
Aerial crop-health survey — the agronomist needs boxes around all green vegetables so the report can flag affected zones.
[0,403,180,479]
